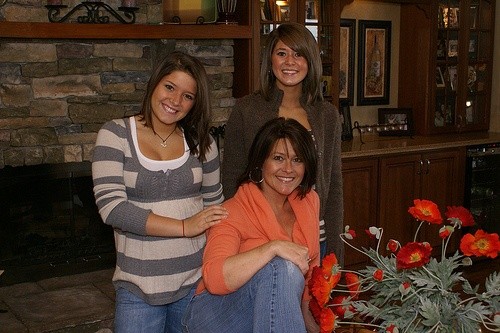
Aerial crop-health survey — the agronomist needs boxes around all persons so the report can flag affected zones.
[180,117,320,333]
[92,50,229,333]
[222,22,345,270]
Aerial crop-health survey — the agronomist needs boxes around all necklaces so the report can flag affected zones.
[136,114,177,148]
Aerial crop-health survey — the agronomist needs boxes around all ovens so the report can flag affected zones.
[463,143,500,260]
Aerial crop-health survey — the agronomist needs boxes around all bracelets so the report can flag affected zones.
[182,219,184,237]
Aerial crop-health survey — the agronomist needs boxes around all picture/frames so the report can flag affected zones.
[339,18,356,106]
[357,20,392,106]
[340,100,353,141]
[377,108,413,137]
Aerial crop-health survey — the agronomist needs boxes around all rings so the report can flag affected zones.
[205,218,208,223]
[307,259,311,263]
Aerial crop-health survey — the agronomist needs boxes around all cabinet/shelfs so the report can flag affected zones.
[0,160,117,288]
[231,0,340,111]
[398,0,495,137]
[341,145,467,278]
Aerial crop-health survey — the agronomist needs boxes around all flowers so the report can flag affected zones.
[308,199,500,333]
